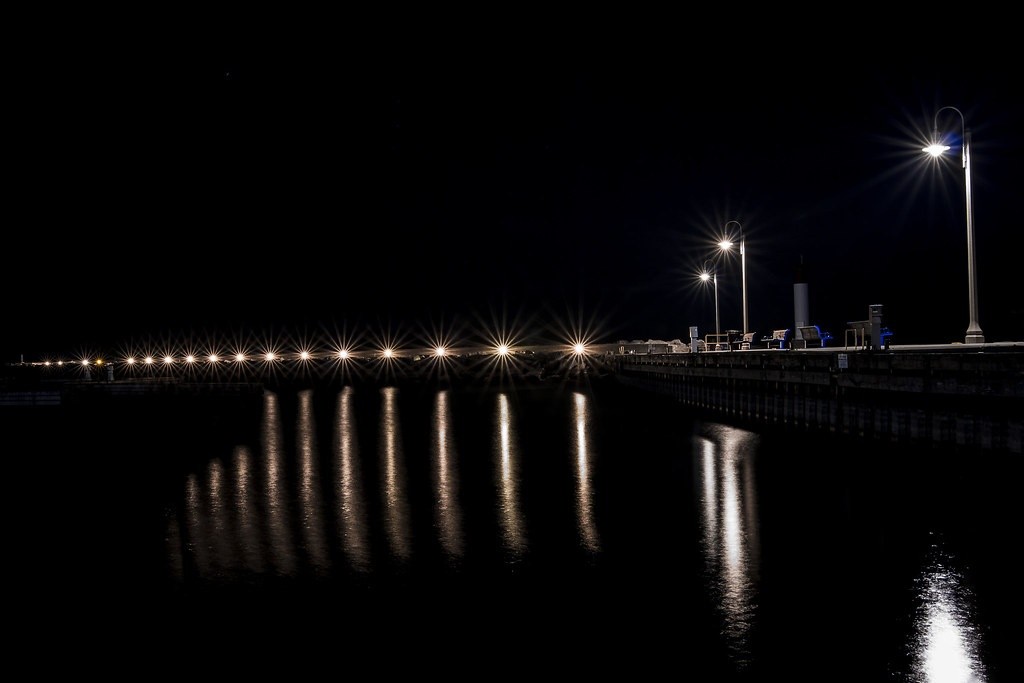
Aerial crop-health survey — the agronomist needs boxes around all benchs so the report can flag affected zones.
[705,321,894,354]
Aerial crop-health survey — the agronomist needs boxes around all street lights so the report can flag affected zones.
[922,107,984,344]
[700,259,722,351]
[718,221,749,350]
